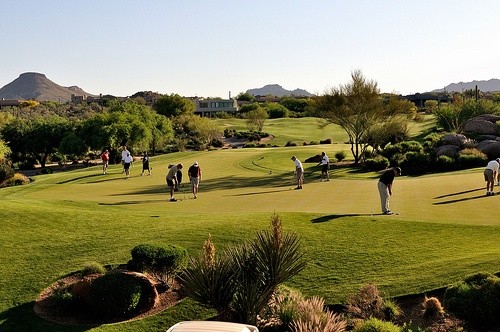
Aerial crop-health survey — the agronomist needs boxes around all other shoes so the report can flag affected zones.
[383,210,393,215]
[170,198,177,201]
[194,196,197,199]
[295,186,302,189]
[487,192,495,195]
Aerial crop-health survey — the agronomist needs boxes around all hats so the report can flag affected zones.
[194,161,198,167]
[395,167,401,176]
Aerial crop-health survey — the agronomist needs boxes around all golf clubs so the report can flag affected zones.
[288,172,294,190]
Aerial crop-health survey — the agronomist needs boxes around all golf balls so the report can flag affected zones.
[396,212,400,215]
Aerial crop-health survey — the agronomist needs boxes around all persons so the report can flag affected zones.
[102,149,109,175]
[166,163,182,202]
[140,151,151,176]
[316,152,330,182]
[484,158,500,195]
[187,161,202,199]
[291,156,304,189]
[121,146,133,180]
[377,167,401,215]
